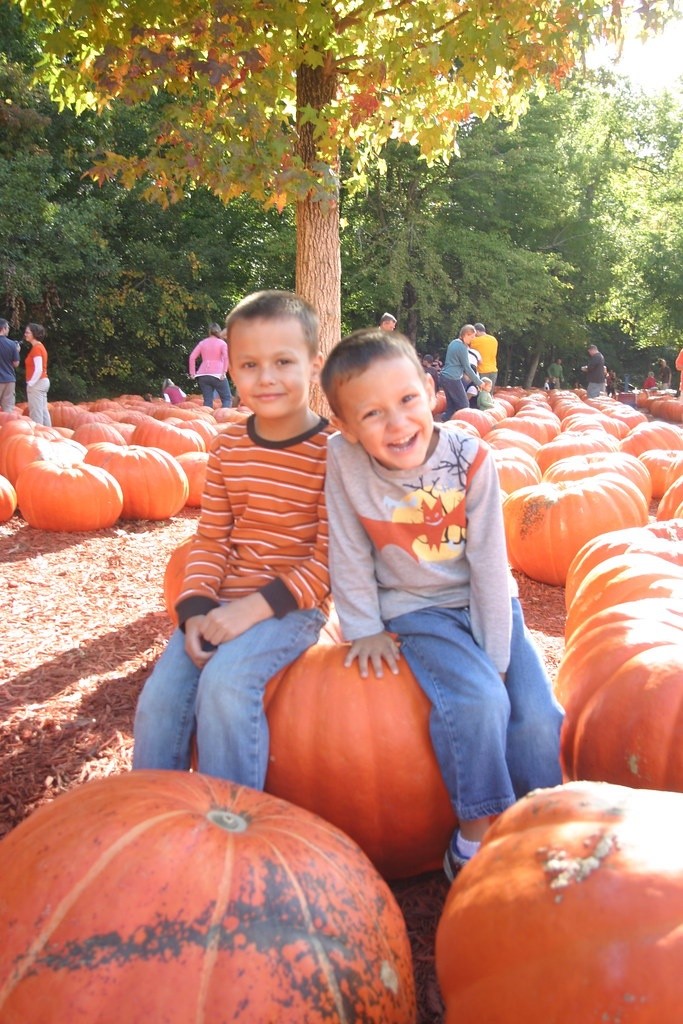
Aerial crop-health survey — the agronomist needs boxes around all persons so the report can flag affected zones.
[644,372,656,389]
[659,359,672,390]
[539,358,563,390]
[675,348,683,404]
[24,323,51,427]
[417,351,444,394]
[603,366,621,398]
[320,331,571,892]
[161,379,185,405]
[0,317,20,412]
[441,321,500,423]
[582,345,604,397]
[189,323,245,409]
[132,290,339,792]
[377,311,396,334]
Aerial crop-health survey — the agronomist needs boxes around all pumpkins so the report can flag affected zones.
[0,385,683,1024]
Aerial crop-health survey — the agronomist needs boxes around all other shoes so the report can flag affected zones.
[443,828,471,883]
